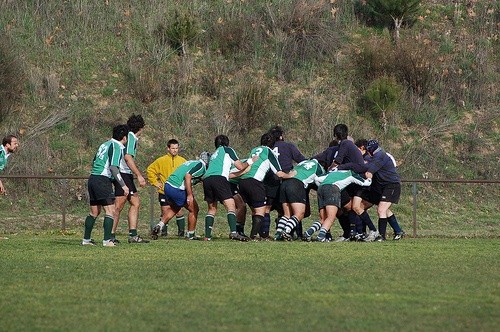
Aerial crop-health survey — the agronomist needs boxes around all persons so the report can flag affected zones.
[285,123,405,241]
[238,132,299,240]
[146,139,189,236]
[82,125,129,247]
[109,114,150,243]
[228,158,250,238]
[260,126,307,239]
[273,153,329,241]
[0,134,18,193]
[151,151,214,240]
[201,135,259,241]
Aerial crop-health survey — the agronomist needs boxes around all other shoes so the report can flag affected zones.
[184,233,200,240]
[151,224,162,240]
[203,236,213,241]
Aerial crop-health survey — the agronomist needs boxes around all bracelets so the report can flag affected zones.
[246,158,253,166]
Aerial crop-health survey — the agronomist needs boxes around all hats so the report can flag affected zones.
[365,139,378,156]
[200,151,212,166]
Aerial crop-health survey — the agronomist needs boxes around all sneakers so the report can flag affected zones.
[111,238,122,245]
[128,234,150,244]
[393,230,405,240]
[102,239,118,247]
[81,238,97,246]
[374,235,388,242]
[228,230,380,242]
